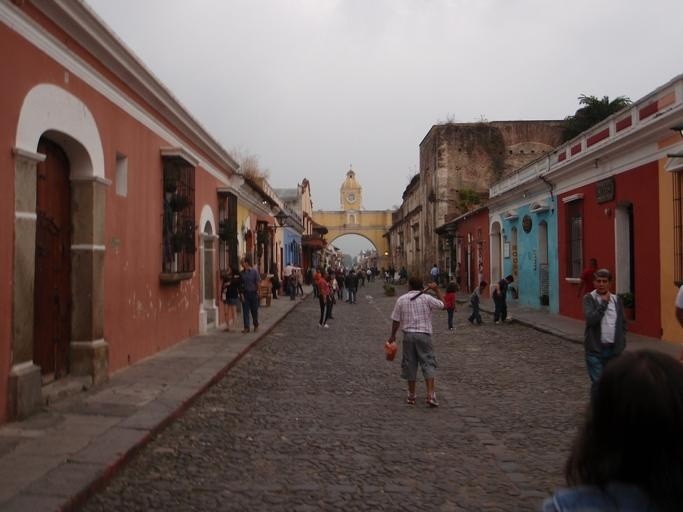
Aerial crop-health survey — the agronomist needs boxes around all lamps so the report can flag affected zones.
[272,209,293,227]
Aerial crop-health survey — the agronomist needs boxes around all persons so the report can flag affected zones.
[388,277,448,406]
[238,258,262,334]
[468,281,487,325]
[492,275,514,324]
[583,268,626,383]
[445,282,458,330]
[431,263,440,286]
[674,285,683,329]
[270,262,406,329]
[220,265,245,331]
[541,349,682,512]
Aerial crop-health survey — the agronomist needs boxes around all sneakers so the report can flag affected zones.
[425,391,441,407]
[241,326,259,334]
[406,390,417,405]
[318,323,329,328]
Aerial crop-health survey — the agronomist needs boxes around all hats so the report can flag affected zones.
[409,277,426,290]
